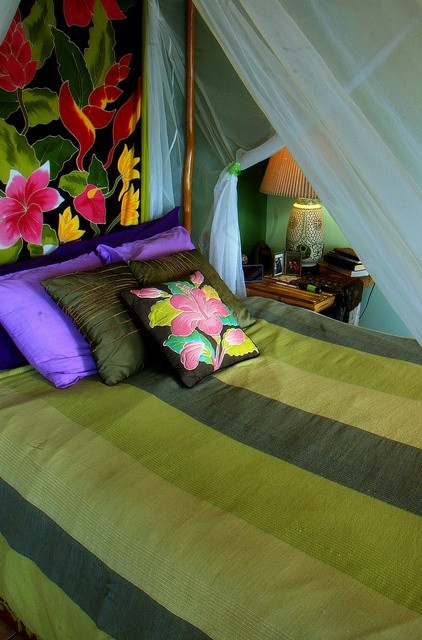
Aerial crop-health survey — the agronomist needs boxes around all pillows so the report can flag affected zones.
[0,206,182,370]
[0,251,102,390]
[40,259,150,386]
[118,268,261,388]
[127,248,257,328]
[95,226,196,266]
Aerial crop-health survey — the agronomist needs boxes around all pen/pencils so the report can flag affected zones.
[276,281,297,288]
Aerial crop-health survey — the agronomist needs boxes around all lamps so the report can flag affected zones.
[259,147,324,268]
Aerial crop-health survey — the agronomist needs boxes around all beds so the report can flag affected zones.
[0,296,421,639]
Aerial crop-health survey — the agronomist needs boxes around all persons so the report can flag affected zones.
[287,260,300,274]
[275,258,282,274]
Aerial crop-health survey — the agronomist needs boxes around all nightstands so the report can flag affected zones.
[245,267,375,313]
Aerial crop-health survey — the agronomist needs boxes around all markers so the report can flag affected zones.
[299,282,323,293]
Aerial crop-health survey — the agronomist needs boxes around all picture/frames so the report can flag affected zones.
[273,253,285,277]
[284,251,301,276]
[242,264,264,283]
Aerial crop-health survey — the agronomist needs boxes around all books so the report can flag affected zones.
[323,247,370,278]
